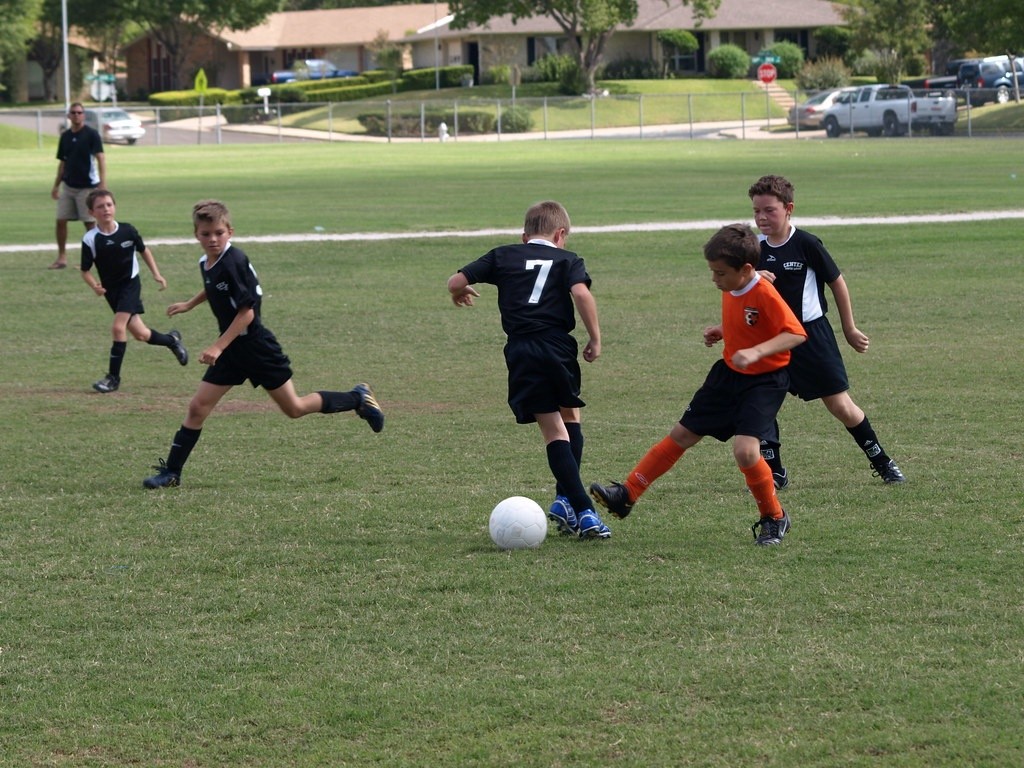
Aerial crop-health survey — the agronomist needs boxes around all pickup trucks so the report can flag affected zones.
[902,58,980,108]
[823,83,958,137]
[254,58,359,86]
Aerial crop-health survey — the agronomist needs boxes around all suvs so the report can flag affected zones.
[956,57,1024,107]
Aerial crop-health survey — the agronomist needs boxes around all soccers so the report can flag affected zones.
[488,496,548,550]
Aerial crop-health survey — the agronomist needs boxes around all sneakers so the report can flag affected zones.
[143,458,180,489]
[590,481,635,521]
[351,381,384,433]
[752,509,791,546]
[870,459,904,484]
[773,468,788,490]
[93,374,121,393]
[548,495,612,540]
[166,330,188,366]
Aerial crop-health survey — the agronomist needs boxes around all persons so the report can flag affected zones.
[746,174,904,494]
[47,102,107,269]
[143,200,383,489]
[80,189,188,392]
[447,201,613,540]
[590,223,807,548]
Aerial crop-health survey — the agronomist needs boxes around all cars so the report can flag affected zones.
[787,85,857,129]
[83,107,146,144]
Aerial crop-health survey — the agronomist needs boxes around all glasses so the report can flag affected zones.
[69,111,83,115]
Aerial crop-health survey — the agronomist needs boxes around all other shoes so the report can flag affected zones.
[47,255,67,270]
